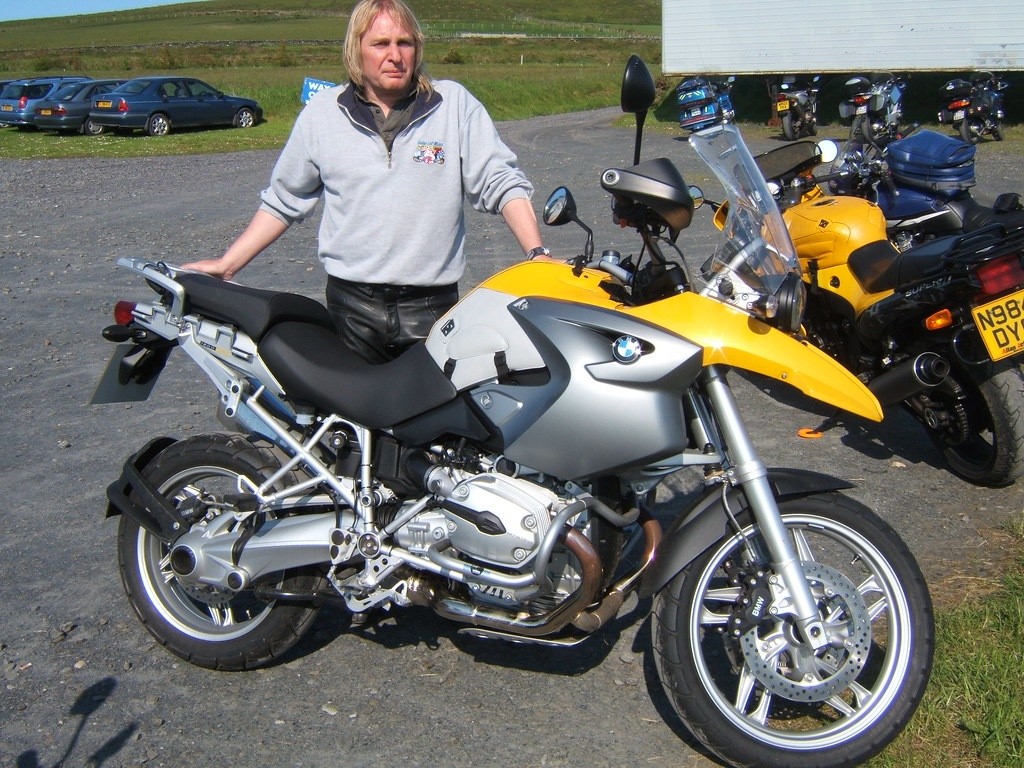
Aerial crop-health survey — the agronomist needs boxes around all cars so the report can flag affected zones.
[35,78,161,134]
[87,74,265,136]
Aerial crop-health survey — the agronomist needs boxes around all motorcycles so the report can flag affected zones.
[935,71,1010,142]
[836,72,920,152]
[689,125,1023,494]
[676,75,738,134]
[765,74,822,140]
[96,55,937,767]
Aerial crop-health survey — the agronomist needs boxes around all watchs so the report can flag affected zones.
[526,246,552,260]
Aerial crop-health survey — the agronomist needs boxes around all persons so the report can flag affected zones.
[179,0,568,624]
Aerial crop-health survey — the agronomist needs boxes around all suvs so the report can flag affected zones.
[0,76,111,132]
